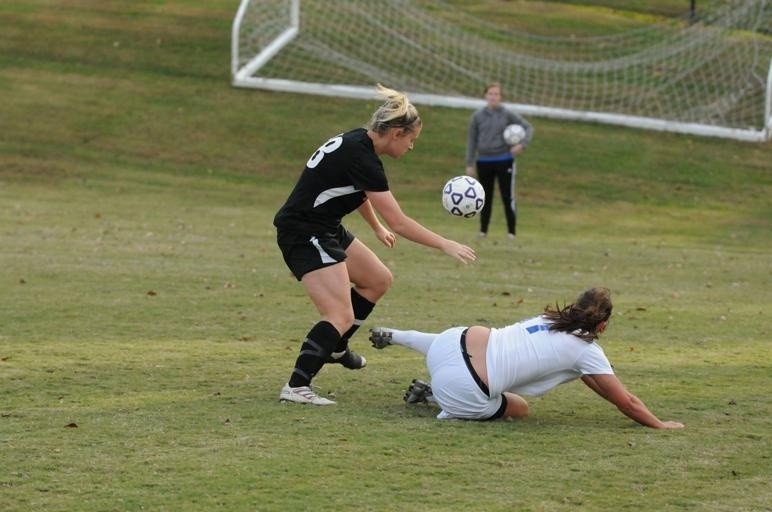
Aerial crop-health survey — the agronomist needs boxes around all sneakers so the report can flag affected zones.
[279,383,336,406]
[403,379,432,402]
[325,347,366,370]
[369,328,392,348]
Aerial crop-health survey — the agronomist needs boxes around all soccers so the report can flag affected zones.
[503,124,526,146]
[443,176,485,218]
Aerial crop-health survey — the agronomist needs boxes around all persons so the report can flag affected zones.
[273,82,477,406]
[369,286,685,429]
[467,82,534,239]
